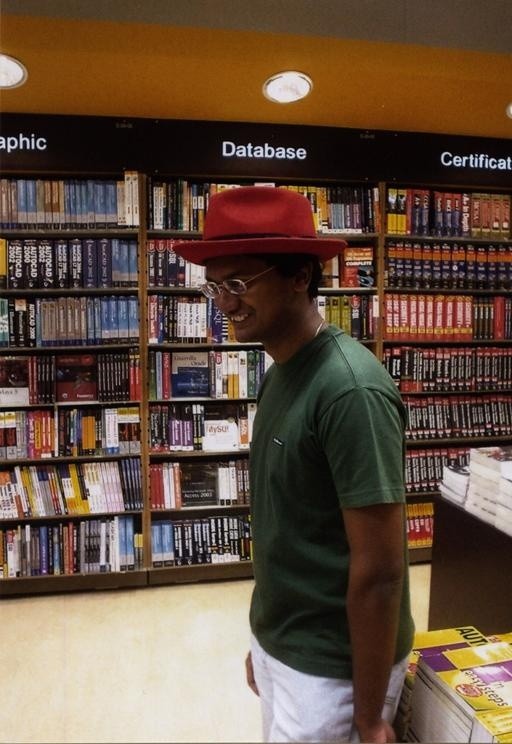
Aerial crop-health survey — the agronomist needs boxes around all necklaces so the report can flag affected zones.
[315,319,326,337]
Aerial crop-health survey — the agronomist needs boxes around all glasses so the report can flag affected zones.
[199,266,275,300]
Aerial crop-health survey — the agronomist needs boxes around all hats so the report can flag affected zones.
[171,186,347,264]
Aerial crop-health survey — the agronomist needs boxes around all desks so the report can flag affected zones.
[421,489,512,637]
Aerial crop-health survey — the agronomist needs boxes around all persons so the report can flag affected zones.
[164,185,417,743]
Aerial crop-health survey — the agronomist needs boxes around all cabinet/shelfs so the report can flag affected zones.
[0,165,147,597]
[140,166,382,594]
[373,173,512,568]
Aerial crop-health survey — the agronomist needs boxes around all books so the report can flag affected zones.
[3,238,138,290]
[311,294,379,341]
[1,171,140,231]
[147,350,274,400]
[3,459,143,518]
[382,347,512,392]
[147,294,236,344]
[151,513,252,568]
[391,440,512,743]
[385,188,511,239]
[150,458,251,508]
[401,394,512,440]
[3,347,141,407]
[384,291,512,341]
[3,295,140,348]
[3,515,144,580]
[147,239,207,288]
[3,408,140,460]
[318,247,375,287]
[148,401,258,451]
[146,176,380,234]
[385,236,512,289]
